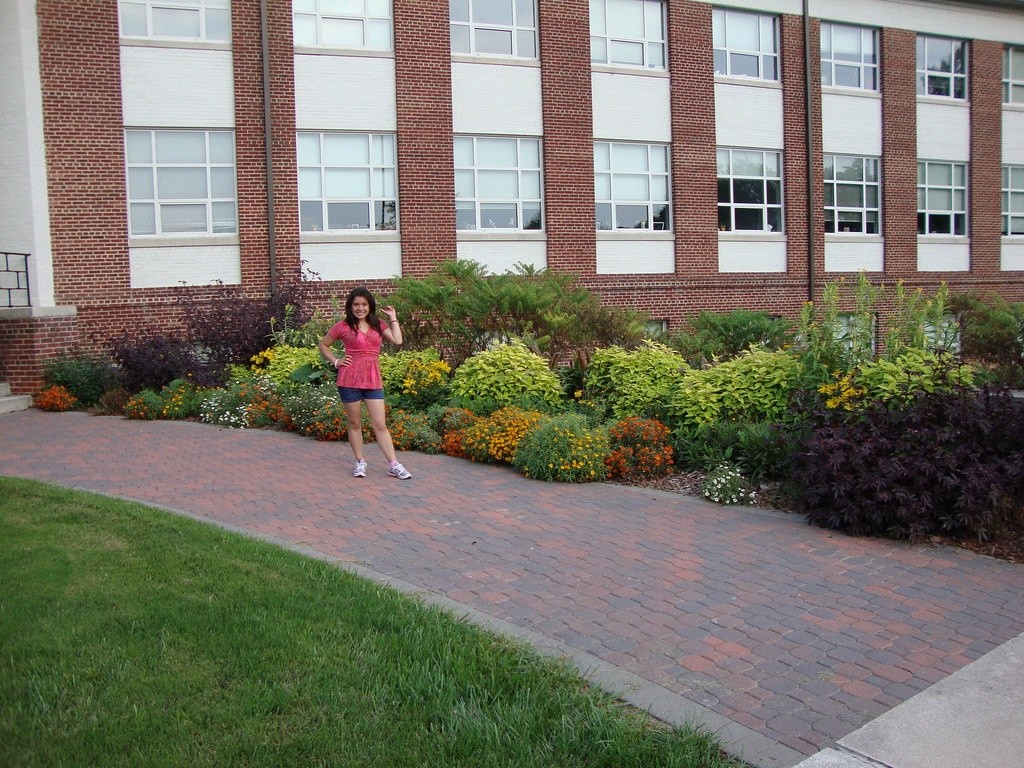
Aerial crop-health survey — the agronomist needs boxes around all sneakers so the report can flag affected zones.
[388,462,411,480]
[353,459,368,477]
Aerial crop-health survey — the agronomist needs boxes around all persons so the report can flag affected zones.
[319,287,411,480]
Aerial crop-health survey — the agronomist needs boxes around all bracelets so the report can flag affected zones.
[335,359,339,369]
[390,320,398,323]
